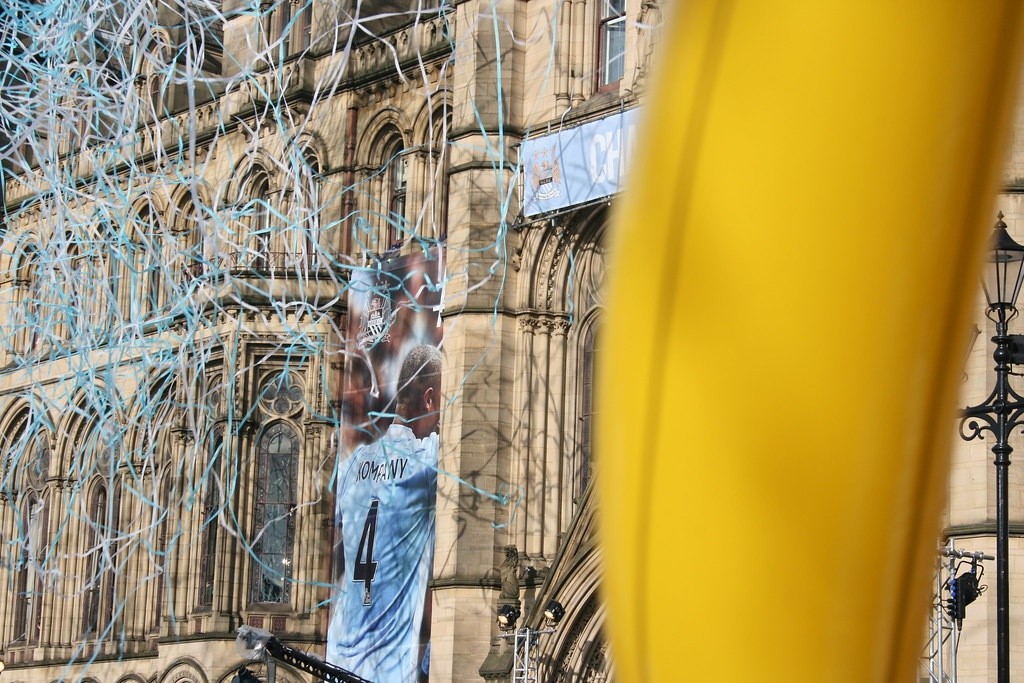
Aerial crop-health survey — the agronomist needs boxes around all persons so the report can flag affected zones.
[323,248,445,683]
